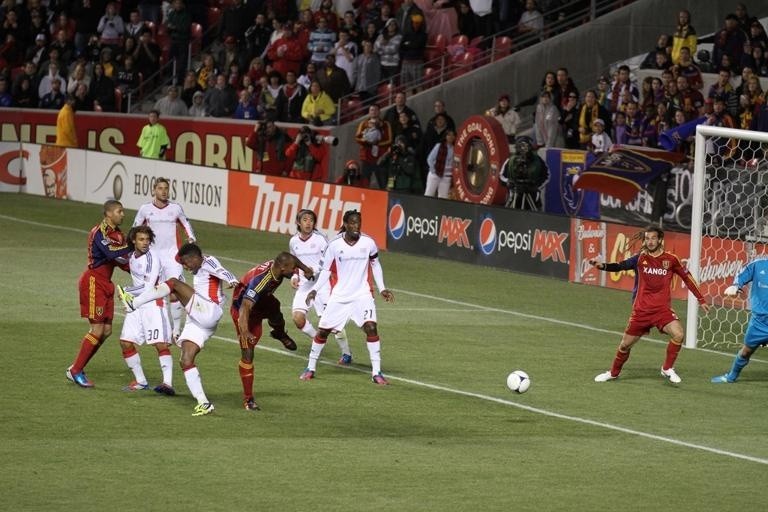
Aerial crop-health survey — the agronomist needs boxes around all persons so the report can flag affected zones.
[130,177,196,347]
[533,4,768,211]
[118,226,175,397]
[228,250,316,414]
[297,207,396,386]
[286,207,354,366]
[1,0,578,211]
[588,224,711,386]
[709,257,768,385]
[114,243,240,418]
[65,195,130,390]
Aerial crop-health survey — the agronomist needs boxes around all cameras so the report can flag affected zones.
[390,145,404,166]
[344,169,356,177]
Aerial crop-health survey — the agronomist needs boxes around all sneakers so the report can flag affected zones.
[661,364,681,385]
[711,374,736,384]
[66,364,95,388]
[242,395,260,411]
[115,284,136,314]
[154,383,175,396]
[371,371,389,385]
[300,367,315,381]
[270,329,297,351]
[191,402,215,417]
[122,382,150,392]
[594,370,621,382]
[337,353,352,366]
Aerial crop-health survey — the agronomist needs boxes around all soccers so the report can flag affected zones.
[508,370,529,393]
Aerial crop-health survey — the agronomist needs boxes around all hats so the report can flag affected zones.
[593,119,604,125]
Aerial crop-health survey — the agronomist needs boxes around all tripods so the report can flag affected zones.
[510,191,539,211]
